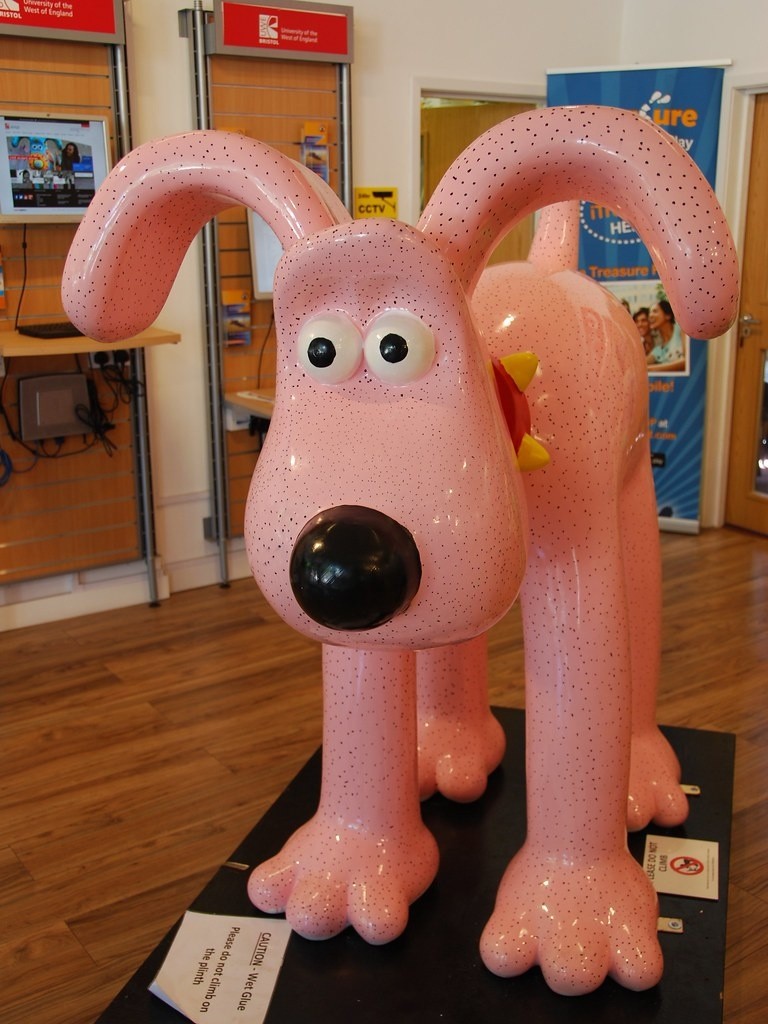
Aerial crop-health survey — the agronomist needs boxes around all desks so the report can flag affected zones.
[0,330,185,627]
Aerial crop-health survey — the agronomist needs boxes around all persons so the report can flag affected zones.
[46,142,81,171]
[620,299,685,371]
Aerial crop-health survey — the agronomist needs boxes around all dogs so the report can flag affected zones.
[60,104,742,995]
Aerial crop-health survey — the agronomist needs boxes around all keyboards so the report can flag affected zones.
[17,322,84,339]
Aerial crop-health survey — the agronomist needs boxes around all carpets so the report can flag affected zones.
[91,707,737,1024]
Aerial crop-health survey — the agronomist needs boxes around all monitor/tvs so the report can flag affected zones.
[0,110,112,223]
